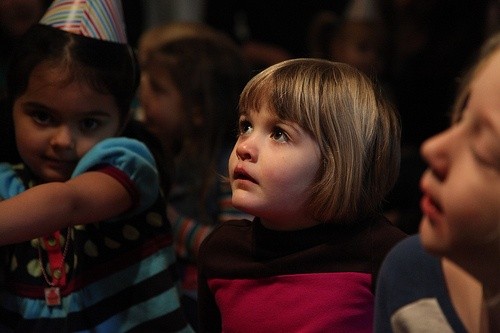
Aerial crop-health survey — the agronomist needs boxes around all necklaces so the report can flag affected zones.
[35,226,72,306]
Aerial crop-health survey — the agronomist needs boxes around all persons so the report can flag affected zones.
[372,31,500,332]
[0,0,403,333]
